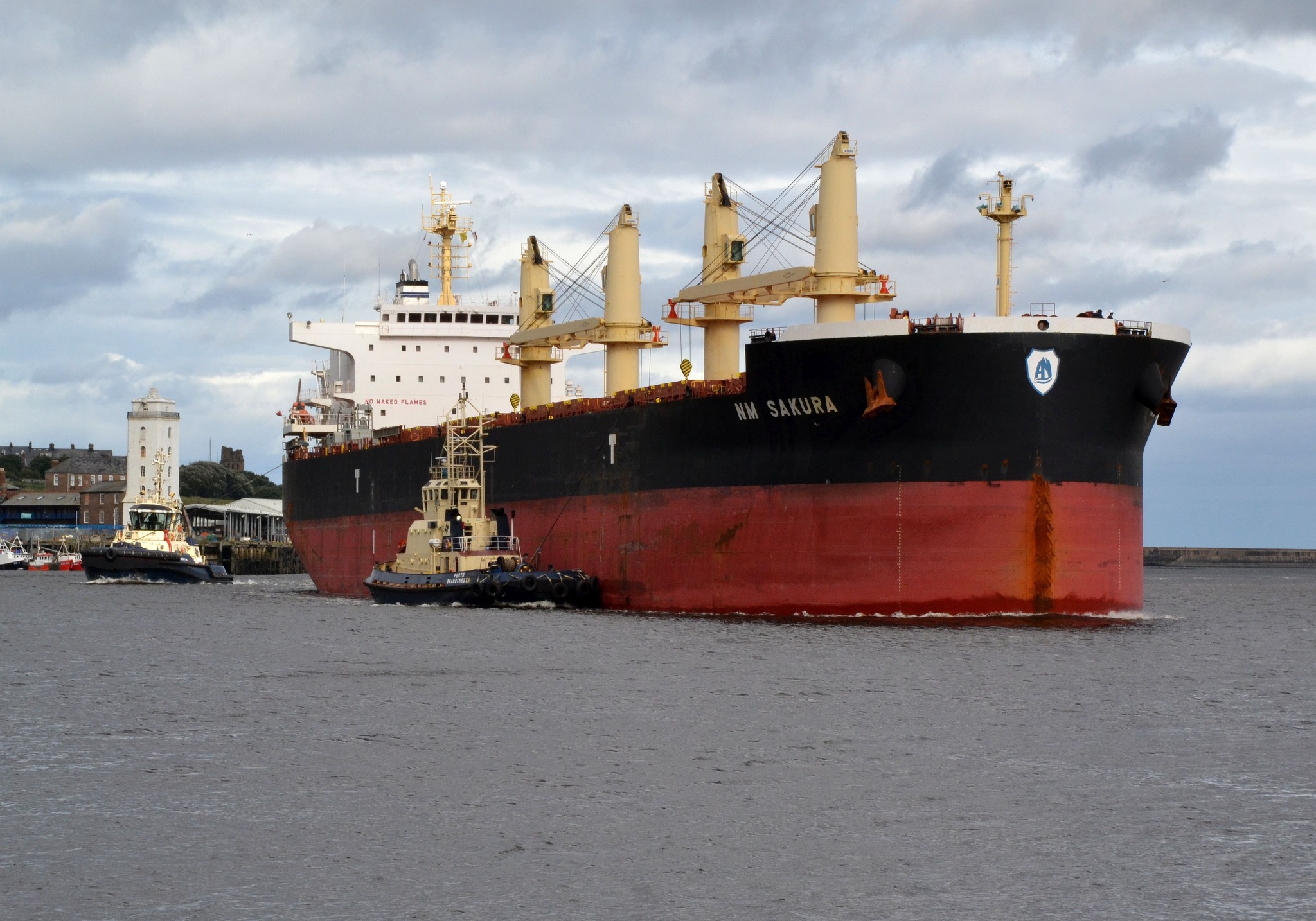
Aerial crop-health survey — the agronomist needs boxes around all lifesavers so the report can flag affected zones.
[120,532,122,539]
[279,548,287,555]
[268,546,273,551]
[470,582,486,599]
[553,582,568,598]
[105,548,116,562]
[590,575,600,592]
[171,534,174,541]
[486,579,504,599]
[522,575,538,591]
[575,580,588,598]
[180,547,184,553]
[307,416,314,424]
[411,433,420,441]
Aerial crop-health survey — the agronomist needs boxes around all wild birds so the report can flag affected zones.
[1158,279,1169,282]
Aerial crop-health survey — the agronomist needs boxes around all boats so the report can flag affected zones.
[80,448,233,583]
[0,532,85,571]
[282,131,1192,621]
[362,383,598,604]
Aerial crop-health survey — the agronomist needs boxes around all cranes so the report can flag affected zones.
[208,438,212,461]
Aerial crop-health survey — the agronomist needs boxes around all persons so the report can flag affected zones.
[1106,311,1113,319]
[1092,309,1104,318]
